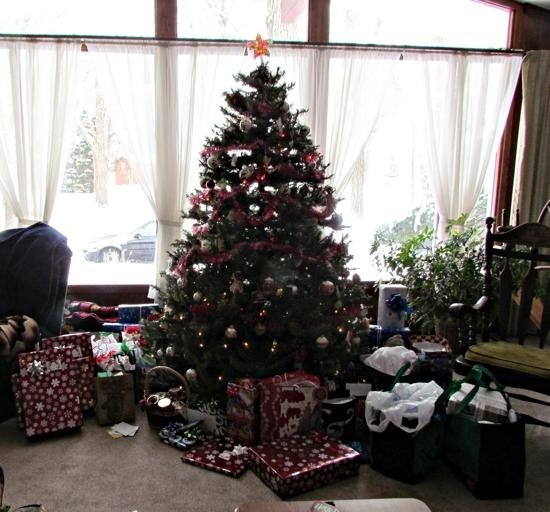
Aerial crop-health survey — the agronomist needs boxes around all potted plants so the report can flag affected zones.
[366,211,545,339]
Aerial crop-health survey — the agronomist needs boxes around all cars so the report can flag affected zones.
[81,219,197,265]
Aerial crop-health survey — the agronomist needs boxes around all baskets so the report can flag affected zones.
[143,366,189,429]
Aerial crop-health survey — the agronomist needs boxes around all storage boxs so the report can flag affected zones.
[144,365,255,479]
[256,342,528,502]
[15,334,136,439]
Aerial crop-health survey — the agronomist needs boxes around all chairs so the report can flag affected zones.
[451,215,550,441]
[0,224,69,420]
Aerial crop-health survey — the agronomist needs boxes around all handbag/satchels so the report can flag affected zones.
[372,362,526,500]
